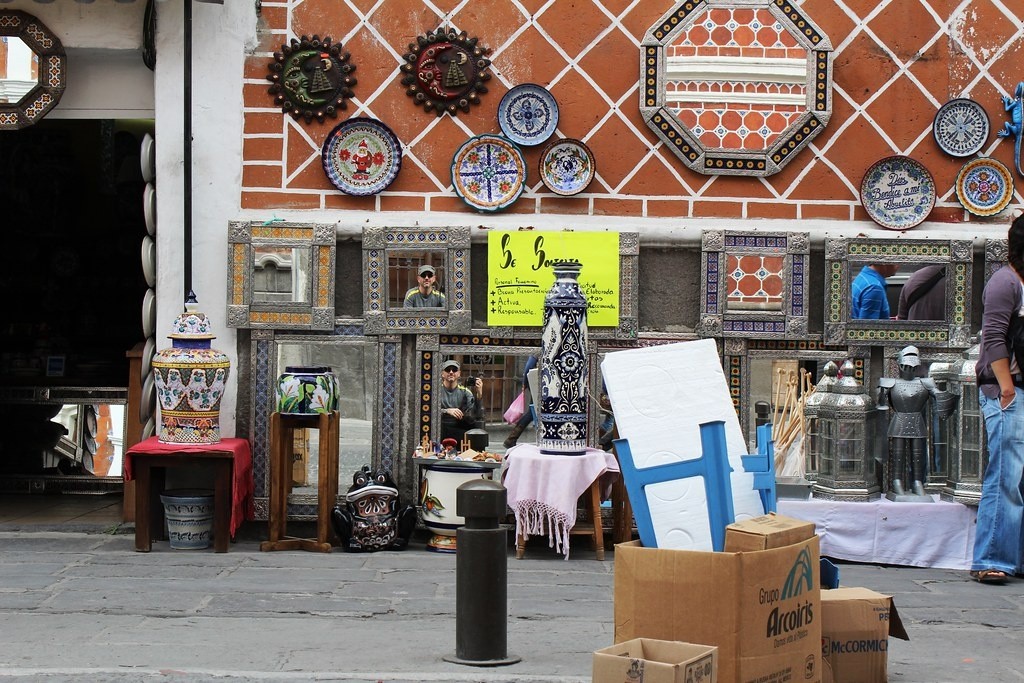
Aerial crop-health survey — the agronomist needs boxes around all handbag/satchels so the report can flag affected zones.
[503,390,527,424]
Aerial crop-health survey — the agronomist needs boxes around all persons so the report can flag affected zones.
[503,355,538,449]
[402,265,445,306]
[852,264,899,319]
[898,265,945,321]
[876,346,942,495]
[970,213,1024,584]
[440,360,485,447]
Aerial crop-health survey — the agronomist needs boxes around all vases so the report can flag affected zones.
[160,489,216,550]
[419,465,494,553]
[539,261,589,455]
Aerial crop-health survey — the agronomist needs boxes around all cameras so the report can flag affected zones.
[465,376,477,386]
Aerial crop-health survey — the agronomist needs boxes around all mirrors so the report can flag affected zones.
[249,318,402,520]
[0,9,67,131]
[701,230,810,341]
[824,238,974,348]
[43,403,85,462]
[1,385,130,496]
[432,346,544,455]
[639,0,833,177]
[722,334,870,499]
[361,225,472,335]
[224,220,336,331]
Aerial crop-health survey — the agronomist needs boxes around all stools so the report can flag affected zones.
[611,420,776,553]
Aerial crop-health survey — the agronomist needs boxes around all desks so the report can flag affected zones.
[123,435,254,554]
[775,495,980,570]
[500,442,621,561]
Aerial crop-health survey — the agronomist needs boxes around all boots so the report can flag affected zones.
[503,424,526,449]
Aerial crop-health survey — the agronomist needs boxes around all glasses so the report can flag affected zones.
[443,367,460,372]
[417,274,435,278]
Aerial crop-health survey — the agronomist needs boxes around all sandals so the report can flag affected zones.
[971,568,1008,582]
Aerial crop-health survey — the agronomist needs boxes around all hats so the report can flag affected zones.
[417,264,436,275]
[441,360,459,371]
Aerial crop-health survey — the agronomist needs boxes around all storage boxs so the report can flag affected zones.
[591,512,910,683]
